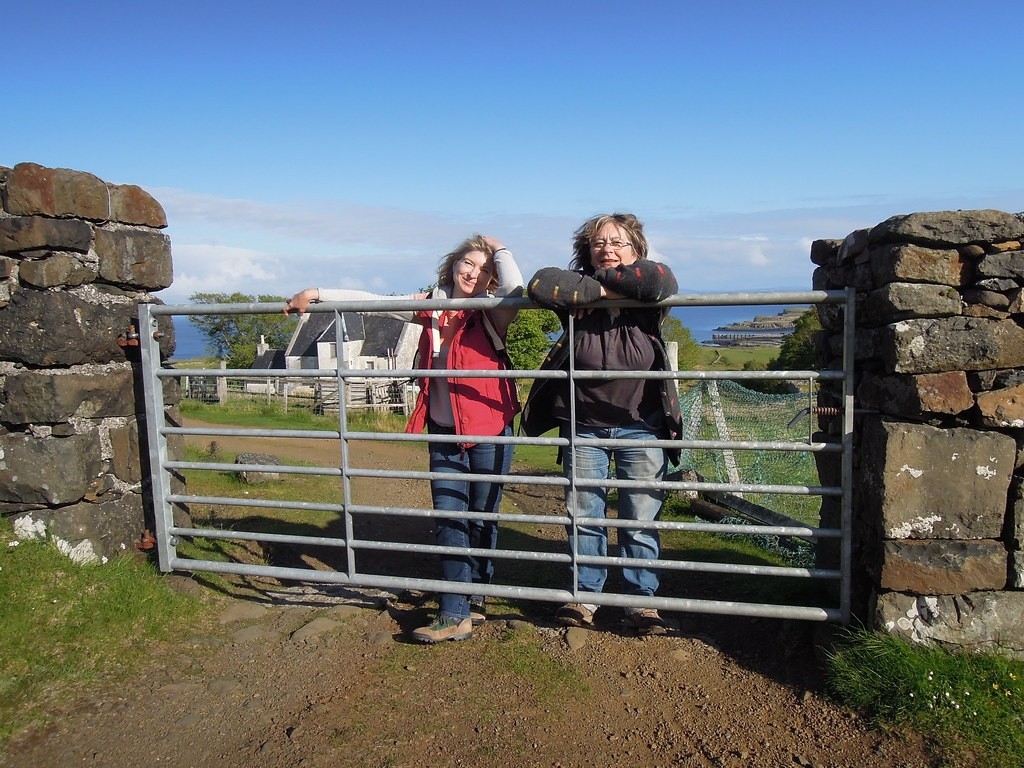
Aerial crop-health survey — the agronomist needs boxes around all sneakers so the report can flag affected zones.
[412,614,472,644]
[555,602,593,627]
[624,607,667,635]
[470,601,486,625]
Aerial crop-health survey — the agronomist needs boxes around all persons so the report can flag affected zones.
[522,215,680,638]
[284,236,524,643]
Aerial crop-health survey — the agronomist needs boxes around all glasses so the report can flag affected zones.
[590,238,633,251]
